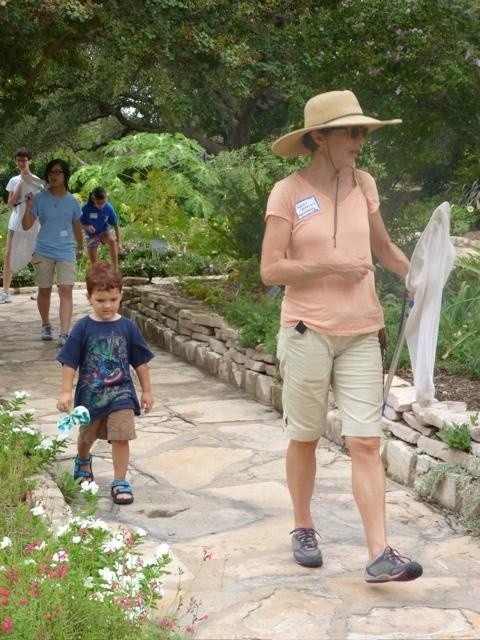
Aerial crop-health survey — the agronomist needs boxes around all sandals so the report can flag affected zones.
[111,480,133,504]
[74,454,94,486]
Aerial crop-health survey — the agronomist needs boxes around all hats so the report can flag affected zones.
[271,90,402,156]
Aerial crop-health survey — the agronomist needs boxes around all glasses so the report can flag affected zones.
[16,159,28,162]
[332,125,368,139]
[48,170,63,176]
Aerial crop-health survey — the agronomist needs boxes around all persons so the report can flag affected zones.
[56,263,154,504]
[0,149,48,304]
[79,187,126,273]
[22,158,84,346]
[259,91,423,583]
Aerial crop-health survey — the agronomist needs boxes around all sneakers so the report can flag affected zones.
[31,293,38,300]
[365,546,423,583]
[58,334,69,346]
[41,324,53,340]
[0,292,11,303]
[289,527,323,567]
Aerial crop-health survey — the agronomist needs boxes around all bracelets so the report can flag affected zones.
[76,249,83,255]
